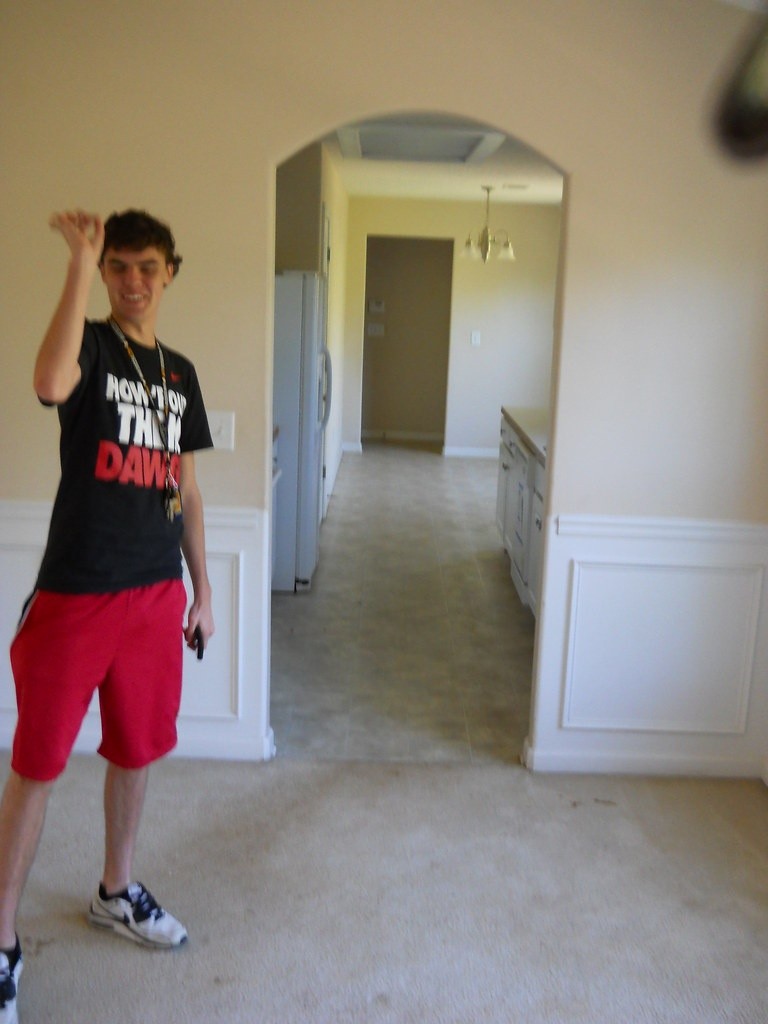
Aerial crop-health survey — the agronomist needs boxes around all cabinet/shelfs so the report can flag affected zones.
[491,407,548,622]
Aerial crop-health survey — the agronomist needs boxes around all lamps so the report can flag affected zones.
[457,184,517,263]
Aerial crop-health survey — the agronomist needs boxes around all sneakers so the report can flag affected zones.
[0,932,23,1024]
[87,880,188,948]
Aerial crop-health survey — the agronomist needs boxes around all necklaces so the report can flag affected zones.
[109,323,184,522]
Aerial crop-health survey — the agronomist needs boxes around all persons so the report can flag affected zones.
[0,206,216,1022]
